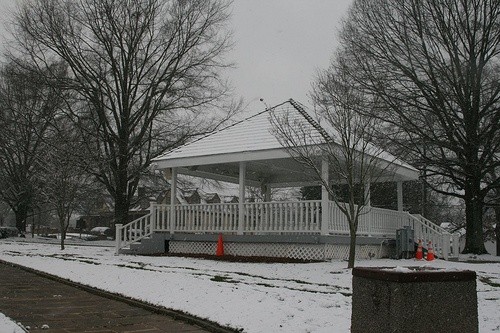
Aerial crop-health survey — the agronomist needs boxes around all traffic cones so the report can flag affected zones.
[427,241,434,261]
[217,232,224,256]
[416,240,423,259]
